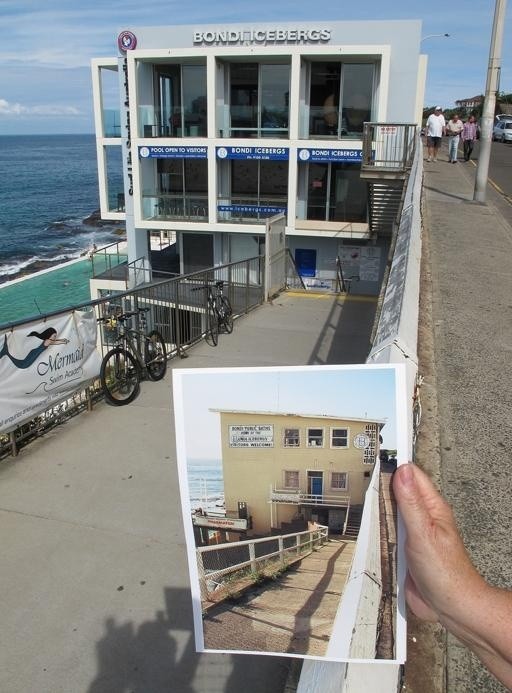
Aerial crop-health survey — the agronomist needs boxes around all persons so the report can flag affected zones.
[392,460,512,693]
[446,113,464,163]
[460,115,478,160]
[424,105,446,162]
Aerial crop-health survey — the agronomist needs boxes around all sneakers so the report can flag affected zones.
[427,155,470,164]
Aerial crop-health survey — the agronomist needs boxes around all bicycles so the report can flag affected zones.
[97,307,167,405]
[191,280,233,345]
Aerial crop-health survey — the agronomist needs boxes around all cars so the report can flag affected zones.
[494,119,512,143]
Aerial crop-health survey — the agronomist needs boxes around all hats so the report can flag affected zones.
[435,106,442,111]
